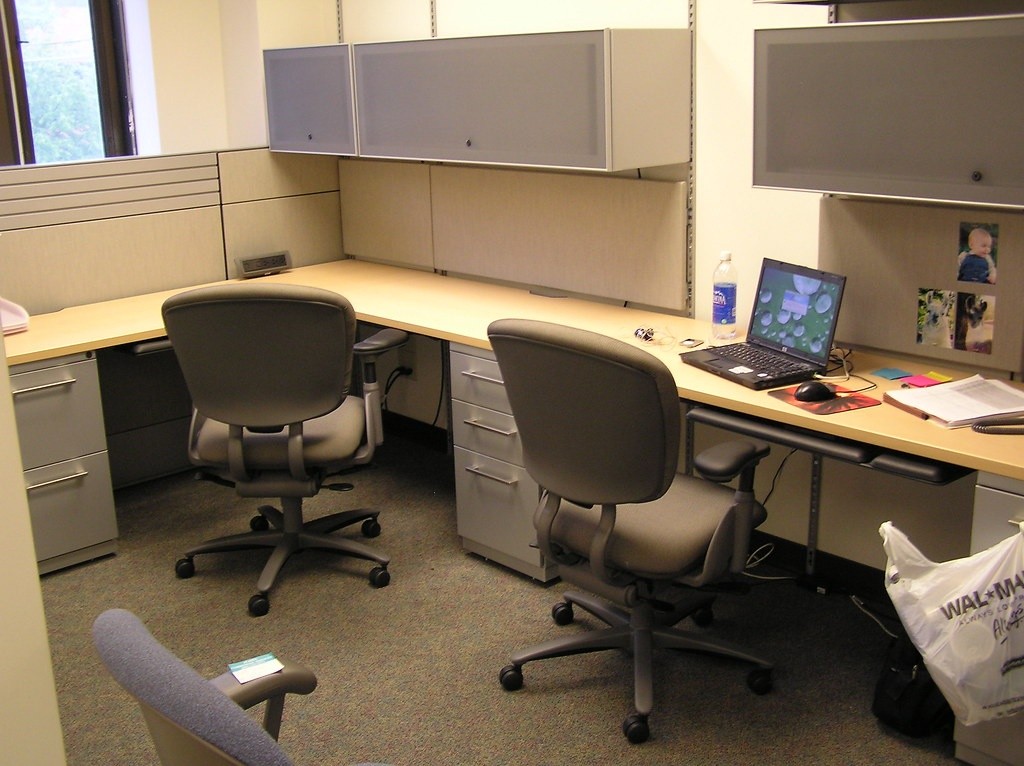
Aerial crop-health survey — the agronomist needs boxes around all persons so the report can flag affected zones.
[955,227,996,284]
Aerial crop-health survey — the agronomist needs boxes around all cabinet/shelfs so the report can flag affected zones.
[753,14,1024,214]
[264,29,690,173]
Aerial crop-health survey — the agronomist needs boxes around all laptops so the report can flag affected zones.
[679,257,847,391]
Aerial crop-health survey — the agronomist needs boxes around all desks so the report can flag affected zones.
[2,258,1024,766]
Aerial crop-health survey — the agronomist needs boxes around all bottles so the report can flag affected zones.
[712,251,737,339]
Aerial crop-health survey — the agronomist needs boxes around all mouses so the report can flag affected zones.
[794,381,836,402]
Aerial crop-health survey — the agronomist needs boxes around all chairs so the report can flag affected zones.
[488,319,775,743]
[162,282,390,618]
[90,607,318,766]
